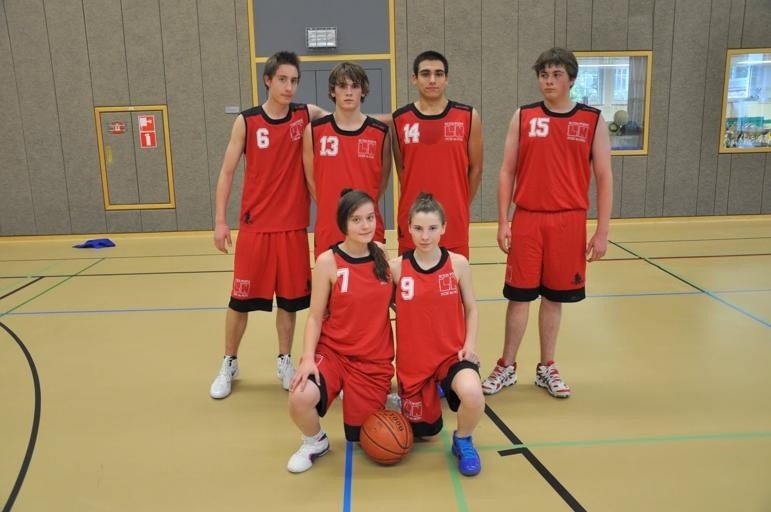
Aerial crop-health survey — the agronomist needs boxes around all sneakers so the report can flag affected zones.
[385,391,402,413]
[449,429,483,478]
[275,353,296,390]
[208,353,241,400]
[481,357,520,396]
[285,431,333,474]
[534,360,573,400]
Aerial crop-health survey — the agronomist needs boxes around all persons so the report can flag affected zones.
[208,51,389,400]
[482,46,616,396]
[304,61,392,262]
[388,194,485,476]
[390,51,484,264]
[284,188,397,474]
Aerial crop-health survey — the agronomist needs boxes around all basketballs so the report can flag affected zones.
[360,409,413,464]
